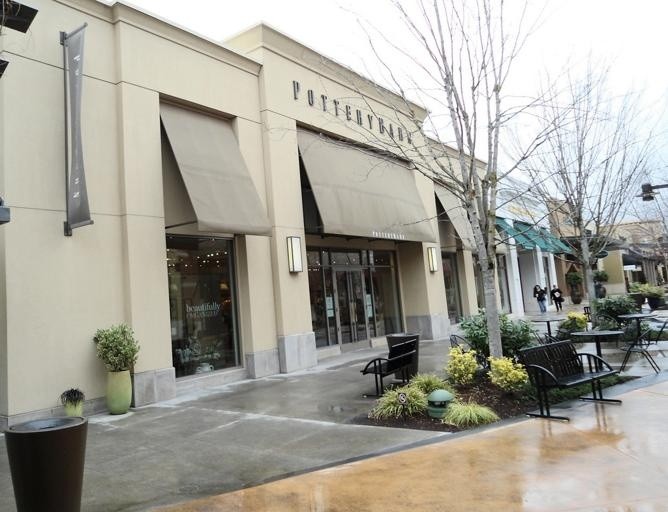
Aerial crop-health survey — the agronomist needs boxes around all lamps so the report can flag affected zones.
[428,247,438,272]
[286,237,302,272]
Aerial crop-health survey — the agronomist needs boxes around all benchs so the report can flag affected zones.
[583,303,602,322]
[517,339,622,421]
[360,339,416,398]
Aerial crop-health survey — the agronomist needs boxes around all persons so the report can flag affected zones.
[549,284,564,312]
[532,285,549,313]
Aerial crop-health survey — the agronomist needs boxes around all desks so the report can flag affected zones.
[531,319,567,344]
[618,313,658,345]
[570,331,624,372]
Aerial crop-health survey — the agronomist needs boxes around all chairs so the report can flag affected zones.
[450,335,480,364]
[555,317,576,341]
[619,319,668,373]
[544,333,560,344]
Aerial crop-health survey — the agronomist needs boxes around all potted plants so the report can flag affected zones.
[60,387,85,419]
[93,320,141,415]
